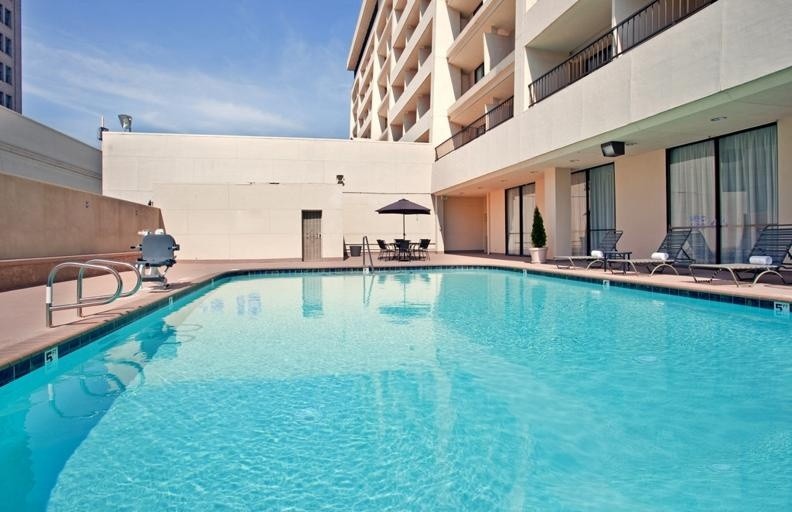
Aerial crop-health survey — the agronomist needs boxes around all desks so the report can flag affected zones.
[604,251,632,271]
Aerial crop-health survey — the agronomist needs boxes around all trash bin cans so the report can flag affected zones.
[350,246,361,256]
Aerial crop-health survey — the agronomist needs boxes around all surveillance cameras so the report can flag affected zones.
[336,174,345,181]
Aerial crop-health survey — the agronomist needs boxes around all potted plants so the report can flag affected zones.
[528,206,548,264]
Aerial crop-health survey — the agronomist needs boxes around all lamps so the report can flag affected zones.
[601,142,625,157]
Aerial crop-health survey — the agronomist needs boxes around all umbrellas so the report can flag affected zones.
[377,274,430,317]
[374,198,431,258]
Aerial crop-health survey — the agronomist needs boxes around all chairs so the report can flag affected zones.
[606,226,696,277]
[688,223,792,288]
[552,230,623,271]
[129,233,180,290]
[376,238,431,262]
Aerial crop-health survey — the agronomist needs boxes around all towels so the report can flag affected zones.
[651,252,670,260]
[591,250,604,258]
[749,255,772,265]
